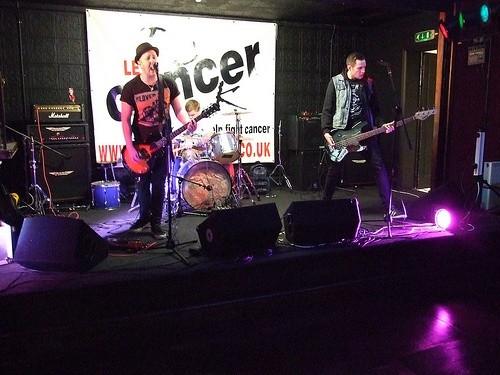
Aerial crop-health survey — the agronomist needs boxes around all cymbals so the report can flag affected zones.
[223,111,252,116]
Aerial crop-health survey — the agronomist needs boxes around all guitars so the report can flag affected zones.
[120,101,222,175]
[324,106,440,164]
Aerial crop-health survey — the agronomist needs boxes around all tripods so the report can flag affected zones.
[218,97,261,207]
[369,67,412,238]
[137,68,199,266]
[0,121,71,215]
[268,119,295,192]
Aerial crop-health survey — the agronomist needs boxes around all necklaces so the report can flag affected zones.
[141,77,154,91]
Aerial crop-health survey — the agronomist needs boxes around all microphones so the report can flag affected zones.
[151,62,158,69]
[377,59,395,67]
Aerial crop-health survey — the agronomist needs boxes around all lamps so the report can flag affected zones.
[434,209,462,230]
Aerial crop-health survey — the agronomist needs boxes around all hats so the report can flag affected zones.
[135,43,159,65]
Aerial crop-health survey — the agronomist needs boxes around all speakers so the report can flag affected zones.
[283,197,362,246]
[195,202,282,259]
[287,114,326,190]
[13,215,110,274]
[22,122,95,208]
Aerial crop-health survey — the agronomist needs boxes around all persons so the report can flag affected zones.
[120,42,198,239]
[321,52,407,218]
[185,100,214,140]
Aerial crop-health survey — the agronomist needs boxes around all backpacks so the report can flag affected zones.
[248,161,271,195]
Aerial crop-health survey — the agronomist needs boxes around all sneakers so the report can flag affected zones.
[151,225,167,240]
[129,218,151,234]
[383,207,407,221]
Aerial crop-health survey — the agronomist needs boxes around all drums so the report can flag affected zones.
[180,146,211,162]
[208,130,240,165]
[91,180,122,210]
[174,159,233,212]
